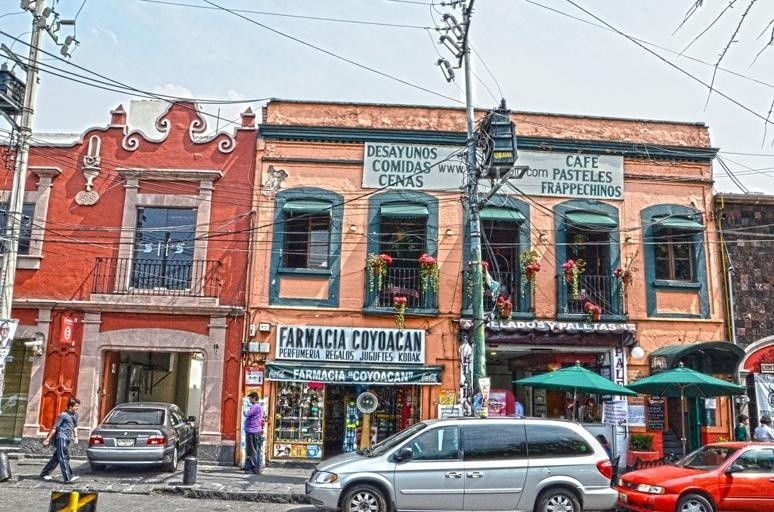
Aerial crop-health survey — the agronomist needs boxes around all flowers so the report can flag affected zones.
[519,250,541,281]
[366,252,391,292]
[417,252,439,294]
[561,257,602,322]
[494,296,514,323]
[613,267,634,290]
[392,296,408,332]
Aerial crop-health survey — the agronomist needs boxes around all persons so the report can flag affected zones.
[240,391,263,474]
[40,398,80,483]
[753,415,774,442]
[276,447,290,457]
[735,415,749,441]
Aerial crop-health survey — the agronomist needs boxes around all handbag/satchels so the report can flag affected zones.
[47,426,56,446]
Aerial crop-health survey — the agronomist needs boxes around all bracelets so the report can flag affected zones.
[74,436,78,437]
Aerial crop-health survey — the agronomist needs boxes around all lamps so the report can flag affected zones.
[629,338,645,360]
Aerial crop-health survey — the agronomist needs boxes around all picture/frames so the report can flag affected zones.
[598,365,612,401]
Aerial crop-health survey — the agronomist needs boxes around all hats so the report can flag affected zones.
[248,392,257,396]
[738,414,749,420]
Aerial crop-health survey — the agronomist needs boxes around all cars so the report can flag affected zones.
[86,401,197,472]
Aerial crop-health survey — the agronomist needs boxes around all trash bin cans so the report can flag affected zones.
[0,450,10,481]
[183,457,197,484]
[219,439,235,466]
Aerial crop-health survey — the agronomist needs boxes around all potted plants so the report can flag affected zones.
[702,436,731,466]
[626,434,660,467]
[570,232,589,256]
[456,330,472,357]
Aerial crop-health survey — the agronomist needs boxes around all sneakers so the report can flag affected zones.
[241,467,260,474]
[43,475,80,484]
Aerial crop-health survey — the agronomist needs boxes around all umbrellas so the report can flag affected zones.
[623,362,750,457]
[512,360,637,422]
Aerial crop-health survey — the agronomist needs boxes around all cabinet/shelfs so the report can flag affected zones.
[275,404,323,433]
